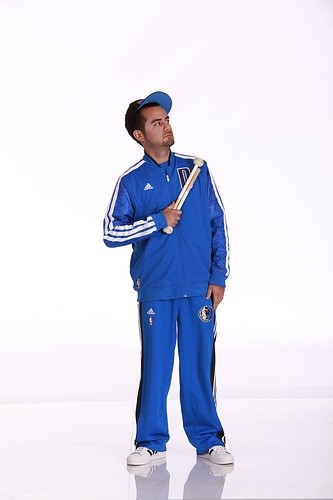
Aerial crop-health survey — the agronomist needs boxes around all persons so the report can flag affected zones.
[101,90,237,466]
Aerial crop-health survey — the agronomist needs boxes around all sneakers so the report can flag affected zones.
[199,445,234,465]
[127,446,166,465]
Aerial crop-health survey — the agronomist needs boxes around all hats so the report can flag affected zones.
[125,91,172,126]
